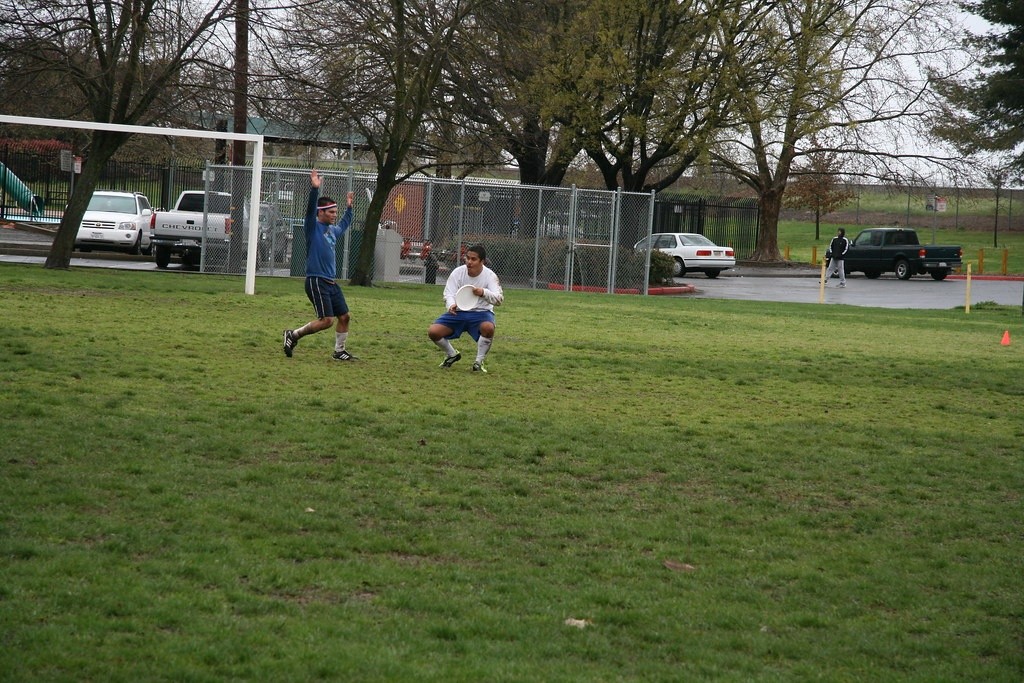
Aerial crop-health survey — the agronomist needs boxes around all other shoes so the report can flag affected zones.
[839,283,846,288]
[819,280,827,283]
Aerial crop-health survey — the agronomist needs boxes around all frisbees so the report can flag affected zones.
[455,285,480,312]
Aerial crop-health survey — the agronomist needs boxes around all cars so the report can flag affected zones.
[65,191,155,255]
[242,204,287,269]
[634,233,736,280]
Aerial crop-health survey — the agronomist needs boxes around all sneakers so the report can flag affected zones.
[439,350,461,369]
[283,330,298,357]
[473,361,487,373]
[332,350,359,362]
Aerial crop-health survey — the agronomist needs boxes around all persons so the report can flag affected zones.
[283,167,360,364]
[428,245,504,374]
[818,227,850,288]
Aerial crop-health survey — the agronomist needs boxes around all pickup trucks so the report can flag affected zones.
[828,228,963,281]
[150,190,260,276]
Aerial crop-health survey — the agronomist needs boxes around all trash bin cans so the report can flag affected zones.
[289,223,374,280]
